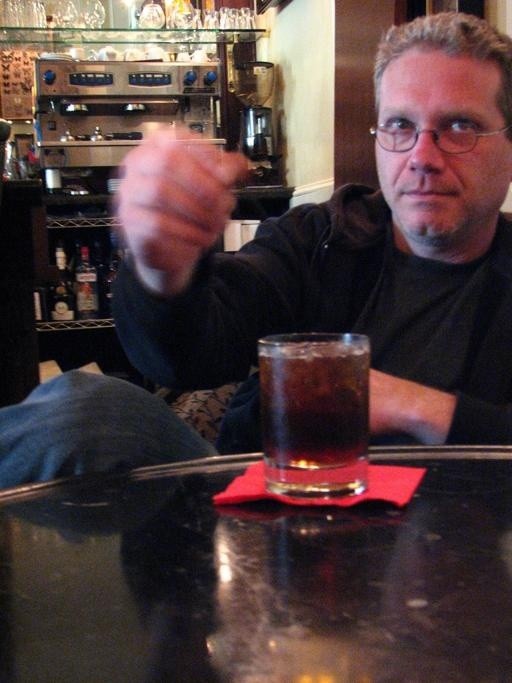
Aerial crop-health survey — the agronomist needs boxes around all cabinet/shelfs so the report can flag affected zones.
[34,185,297,394]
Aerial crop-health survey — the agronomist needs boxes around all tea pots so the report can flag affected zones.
[89,47,120,62]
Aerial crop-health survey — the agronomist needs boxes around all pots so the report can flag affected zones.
[229,189,293,221]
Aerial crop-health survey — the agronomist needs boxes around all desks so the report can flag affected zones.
[1,443,512,683]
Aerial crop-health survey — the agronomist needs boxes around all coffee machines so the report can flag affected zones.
[230,59,275,184]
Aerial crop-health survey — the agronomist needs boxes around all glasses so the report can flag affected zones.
[369,124,509,153]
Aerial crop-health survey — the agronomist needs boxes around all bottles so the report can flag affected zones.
[35,235,126,320]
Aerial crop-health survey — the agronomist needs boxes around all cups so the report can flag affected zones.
[0,0,259,32]
[258,334,367,500]
[191,51,210,61]
[177,53,191,62]
[69,47,84,59]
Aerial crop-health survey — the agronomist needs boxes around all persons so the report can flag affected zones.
[0,13,511,492]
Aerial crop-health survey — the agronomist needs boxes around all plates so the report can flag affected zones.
[45,52,74,61]
[107,178,123,194]
